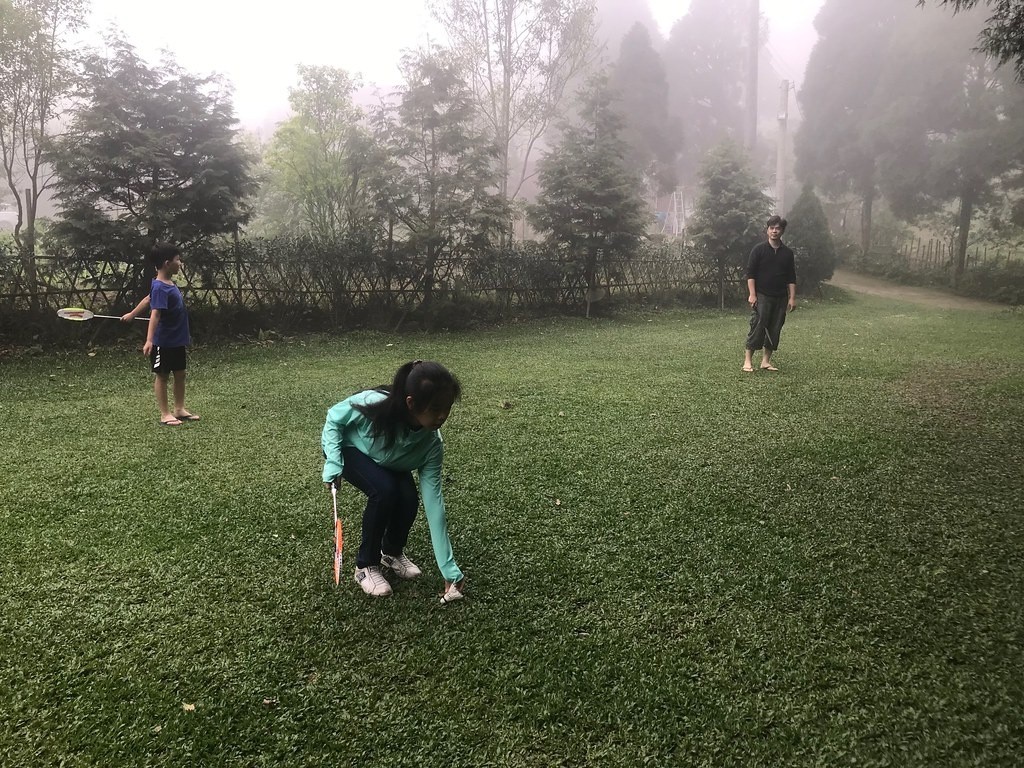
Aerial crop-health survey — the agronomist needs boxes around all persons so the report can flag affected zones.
[743,216,796,372]
[120,242,201,425]
[322,359,465,595]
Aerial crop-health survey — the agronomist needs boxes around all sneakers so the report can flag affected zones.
[354,565,393,597]
[380,550,421,580]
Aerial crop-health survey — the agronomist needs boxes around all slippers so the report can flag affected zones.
[760,365,778,372]
[175,413,200,421]
[159,419,183,426]
[742,367,754,372]
